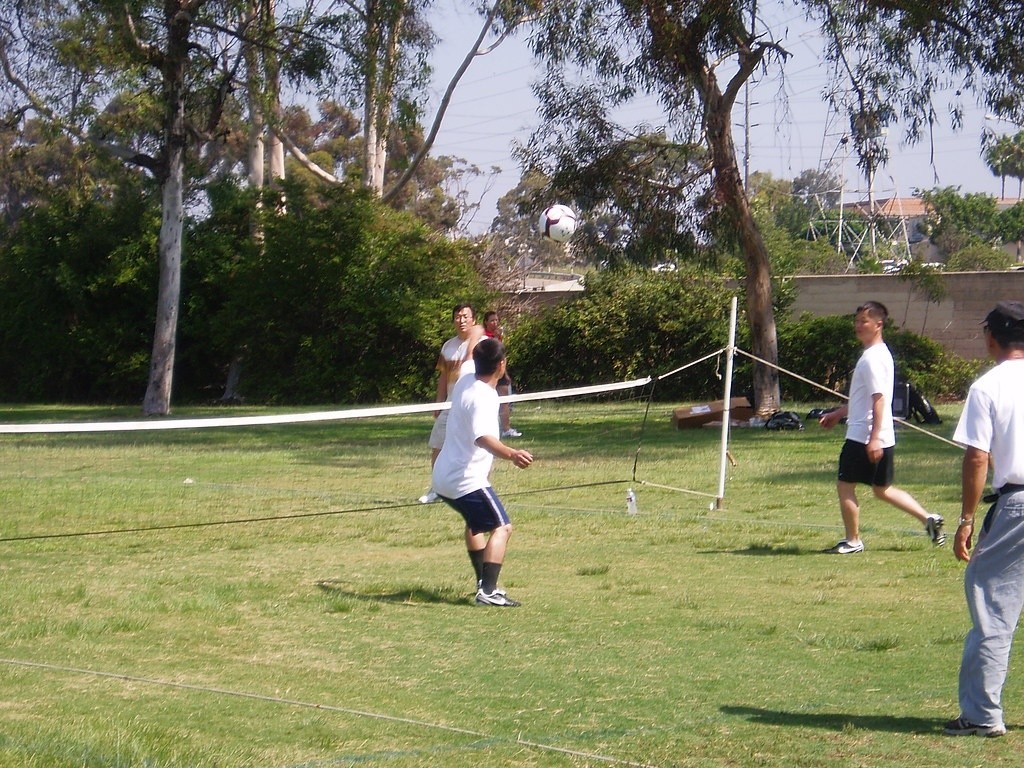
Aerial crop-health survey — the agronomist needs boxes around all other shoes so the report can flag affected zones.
[501,429,522,437]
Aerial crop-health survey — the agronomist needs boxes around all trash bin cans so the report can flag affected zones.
[893,381,910,418]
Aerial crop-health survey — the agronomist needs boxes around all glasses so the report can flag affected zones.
[983,324,992,334]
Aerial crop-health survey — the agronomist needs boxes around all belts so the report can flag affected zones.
[983,483,1024,503]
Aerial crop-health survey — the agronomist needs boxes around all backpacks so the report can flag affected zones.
[806,409,825,419]
[763,411,803,430]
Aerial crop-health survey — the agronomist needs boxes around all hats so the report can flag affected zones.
[977,302,1024,336]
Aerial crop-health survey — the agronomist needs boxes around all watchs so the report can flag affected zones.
[959,517,974,526]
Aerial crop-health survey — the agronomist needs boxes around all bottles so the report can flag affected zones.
[625,488,637,515]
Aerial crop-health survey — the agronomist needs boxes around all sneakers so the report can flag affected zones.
[477,579,482,593]
[418,488,444,504]
[924,514,947,549]
[475,588,521,607]
[944,714,1006,738]
[823,539,864,554]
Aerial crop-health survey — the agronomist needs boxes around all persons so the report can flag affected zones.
[418,305,490,504]
[483,311,522,437]
[433,326,534,607]
[946,301,1023,738]
[818,301,946,552]
